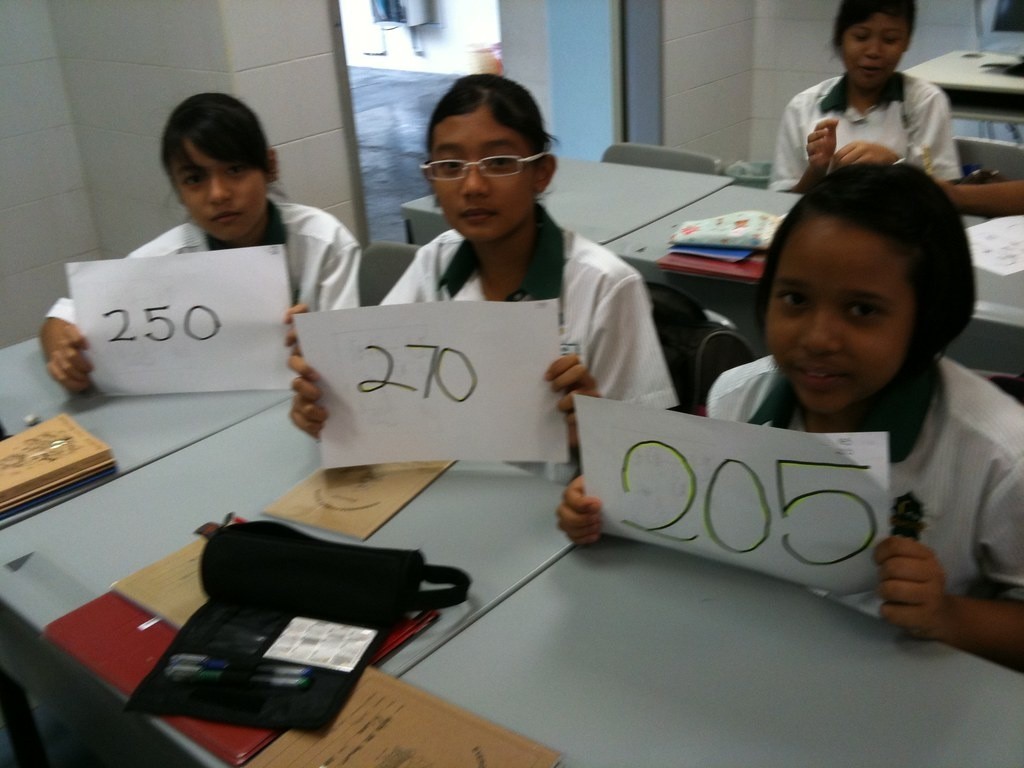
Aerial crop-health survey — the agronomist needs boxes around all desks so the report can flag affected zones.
[902,49,1024,125]
[602,182,1024,380]
[0,337,305,529]
[245,534,1024,768]
[0,391,579,768]
[400,163,735,245]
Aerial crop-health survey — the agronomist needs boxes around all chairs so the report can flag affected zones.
[954,136,1024,181]
[601,142,722,176]
[360,241,422,308]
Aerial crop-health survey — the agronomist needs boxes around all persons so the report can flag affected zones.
[42,90,362,394]
[771,0,1024,222]
[290,75,679,445]
[561,166,1024,677]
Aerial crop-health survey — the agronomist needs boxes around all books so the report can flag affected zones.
[241,668,565,768]
[110,520,237,630]
[263,460,455,541]
[0,413,117,520]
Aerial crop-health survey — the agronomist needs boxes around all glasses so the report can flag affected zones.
[419,150,550,182]
